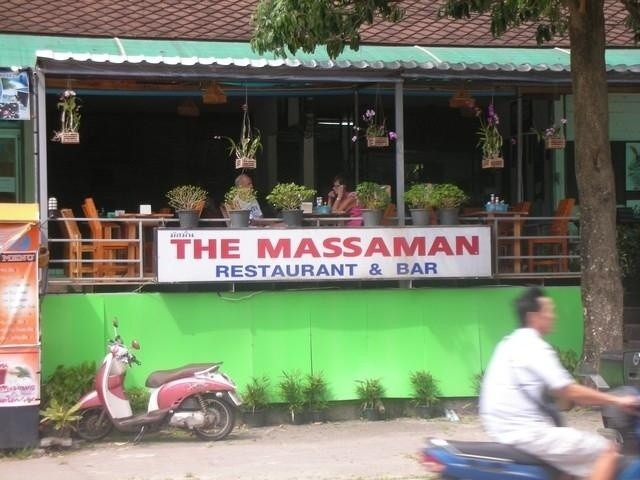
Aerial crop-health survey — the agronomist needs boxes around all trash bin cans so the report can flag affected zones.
[599,346,640,388]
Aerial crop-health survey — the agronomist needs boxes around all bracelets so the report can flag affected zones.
[336,198,342,201]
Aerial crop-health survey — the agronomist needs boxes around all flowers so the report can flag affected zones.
[49,90,83,131]
[529,119,569,139]
[352,109,398,141]
[475,105,516,158]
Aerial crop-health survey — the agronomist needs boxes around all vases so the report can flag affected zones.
[481,158,505,170]
[50,131,81,144]
[365,138,389,149]
[543,139,566,151]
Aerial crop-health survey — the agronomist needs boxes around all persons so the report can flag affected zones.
[477,285,640,480]
[328,175,363,227]
[223,174,266,226]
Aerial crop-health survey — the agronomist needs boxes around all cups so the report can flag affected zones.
[139,203,152,214]
[108,212,115,218]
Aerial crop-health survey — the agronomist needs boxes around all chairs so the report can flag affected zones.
[60,186,576,282]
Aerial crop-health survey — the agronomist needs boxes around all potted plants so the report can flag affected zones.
[274,369,305,425]
[213,103,264,168]
[352,377,386,421]
[301,370,332,424]
[237,375,271,427]
[407,368,442,419]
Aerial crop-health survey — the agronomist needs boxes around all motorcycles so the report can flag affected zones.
[419,362,640,479]
[70,318,242,444]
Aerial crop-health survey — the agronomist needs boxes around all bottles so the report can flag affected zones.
[316,201,328,214]
[485,193,508,212]
[99,208,107,218]
